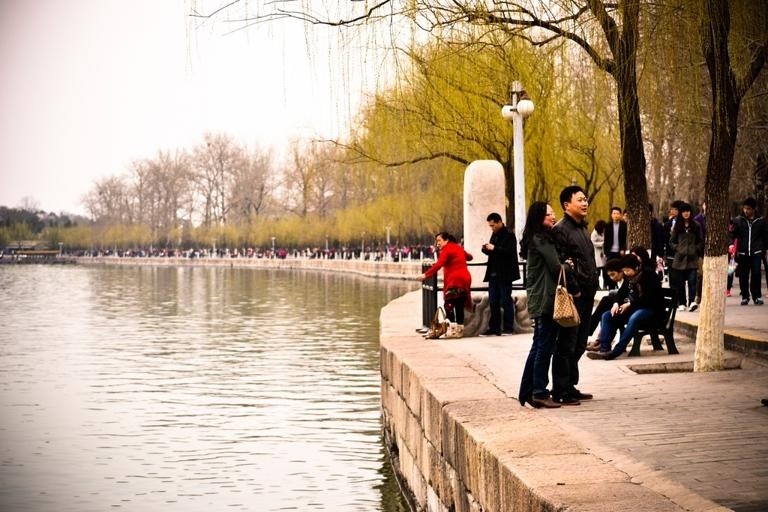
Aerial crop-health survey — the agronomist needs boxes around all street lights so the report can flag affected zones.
[270,236,276,248]
[385,224,393,245]
[57,242,63,256]
[324,234,329,249]
[360,231,366,252]
[211,239,217,252]
[502,93,535,259]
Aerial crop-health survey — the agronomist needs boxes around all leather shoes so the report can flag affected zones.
[519,391,593,409]
[586,341,610,360]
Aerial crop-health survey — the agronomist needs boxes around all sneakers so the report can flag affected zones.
[741,295,750,305]
[501,328,512,334]
[479,329,501,336]
[688,302,698,312]
[754,298,764,304]
[725,290,732,296]
[677,305,686,312]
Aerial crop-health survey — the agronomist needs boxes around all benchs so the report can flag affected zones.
[619,287,681,356]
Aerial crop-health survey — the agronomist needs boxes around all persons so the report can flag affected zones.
[583,184,768,361]
[72,238,466,261]
[416,232,472,339]
[479,212,520,337]
[553,186,600,407]
[519,200,576,410]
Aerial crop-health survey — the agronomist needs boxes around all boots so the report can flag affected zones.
[439,322,465,340]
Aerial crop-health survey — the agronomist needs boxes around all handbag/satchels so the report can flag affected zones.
[428,321,447,339]
[553,285,581,327]
